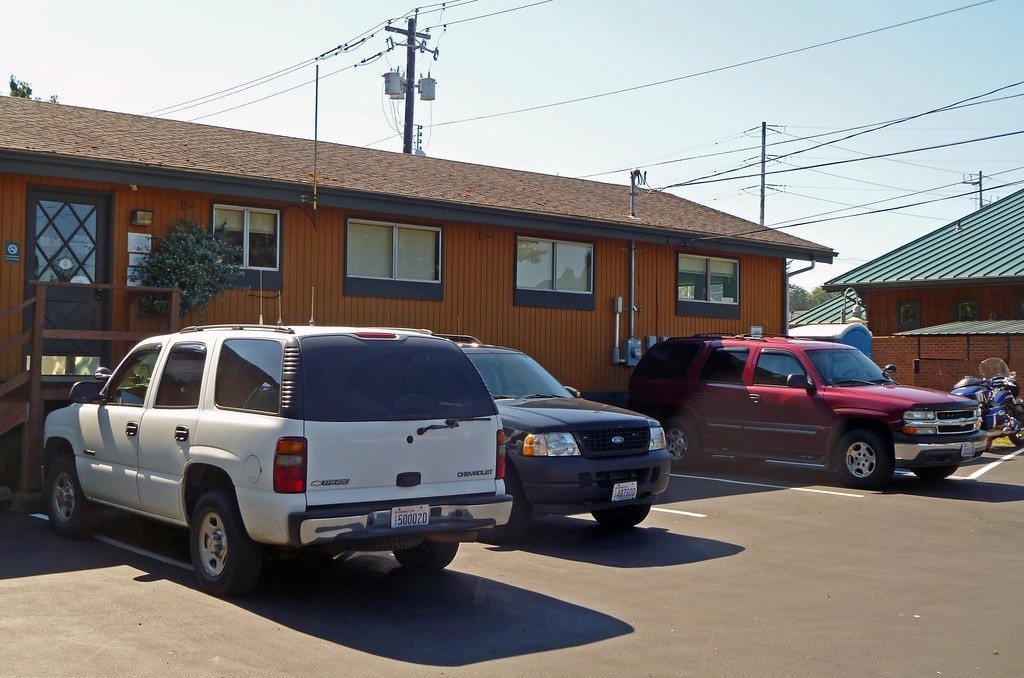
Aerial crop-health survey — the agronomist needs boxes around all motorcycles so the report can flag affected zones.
[948,357,1023,450]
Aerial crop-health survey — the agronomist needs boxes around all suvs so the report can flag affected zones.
[41,321,512,600]
[627,332,987,490]
[435,329,674,550]
